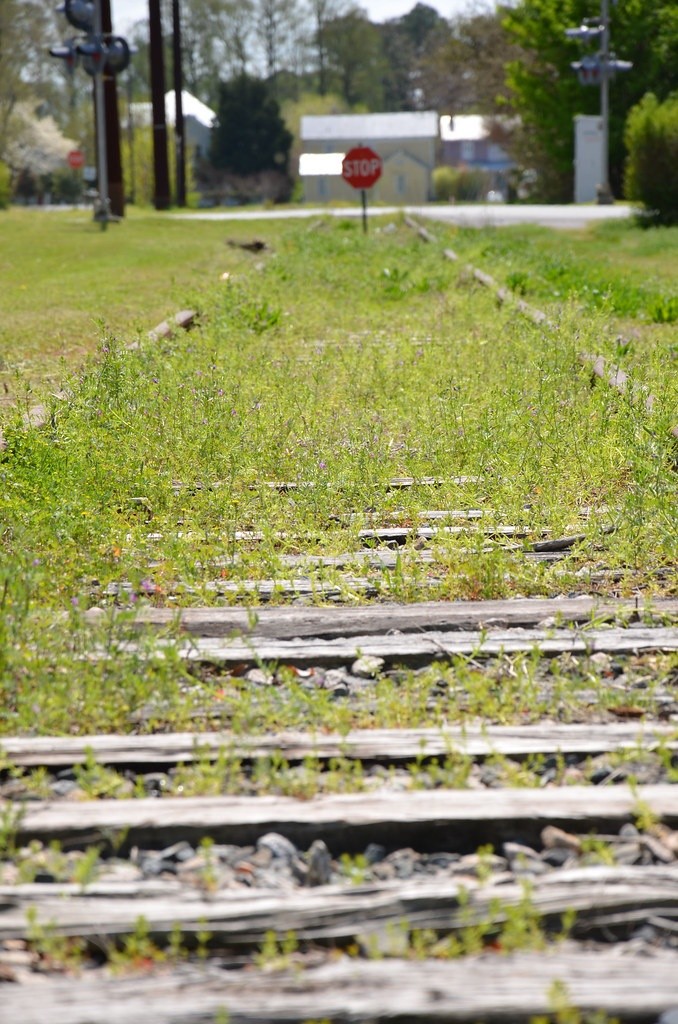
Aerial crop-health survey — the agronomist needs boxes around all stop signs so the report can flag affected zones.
[68,151,84,168]
[342,147,382,189]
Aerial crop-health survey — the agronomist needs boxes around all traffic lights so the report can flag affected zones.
[48,38,107,73]
[571,59,606,85]
